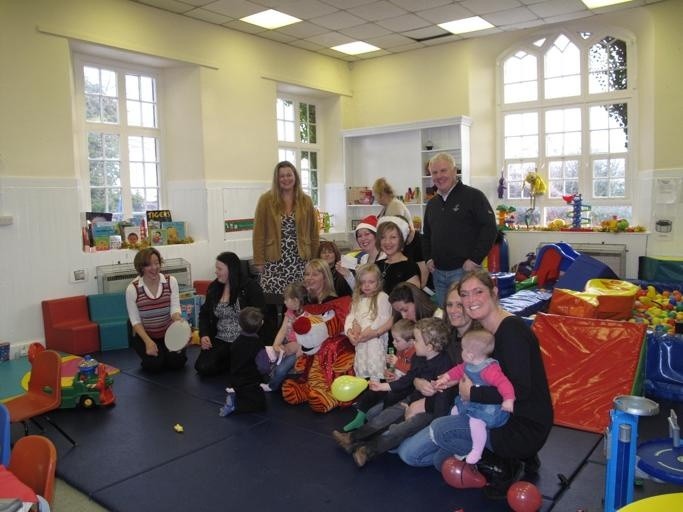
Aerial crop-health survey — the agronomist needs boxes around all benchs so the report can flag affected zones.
[500,286,553,317]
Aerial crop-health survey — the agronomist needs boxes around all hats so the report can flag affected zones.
[354,216,377,236]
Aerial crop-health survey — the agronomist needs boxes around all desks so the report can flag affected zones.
[1,464,39,512]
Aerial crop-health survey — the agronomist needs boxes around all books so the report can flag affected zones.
[82,210,185,251]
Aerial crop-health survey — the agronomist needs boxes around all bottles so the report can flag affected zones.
[78,355,99,390]
[109,236,122,249]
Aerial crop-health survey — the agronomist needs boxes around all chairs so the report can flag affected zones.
[89,291,133,353]
[0,402,11,470]
[6,434,57,504]
[42,295,101,355]
[2,350,77,446]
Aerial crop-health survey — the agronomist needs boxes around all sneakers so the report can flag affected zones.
[356,447,375,466]
[526,454,540,472]
[487,462,524,499]
[333,431,353,454]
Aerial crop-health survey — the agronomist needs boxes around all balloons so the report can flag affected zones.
[507,480,543,512]
[441,456,490,489]
[164,319,192,352]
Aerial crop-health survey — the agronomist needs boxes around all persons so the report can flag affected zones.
[253,161,320,331]
[125,248,188,378]
[332,281,483,467]
[396,268,554,501]
[219,307,271,418]
[422,151,498,310]
[436,328,516,464]
[257,176,457,456]
[194,252,267,377]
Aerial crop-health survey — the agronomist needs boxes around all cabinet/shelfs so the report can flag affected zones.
[340,114,474,250]
